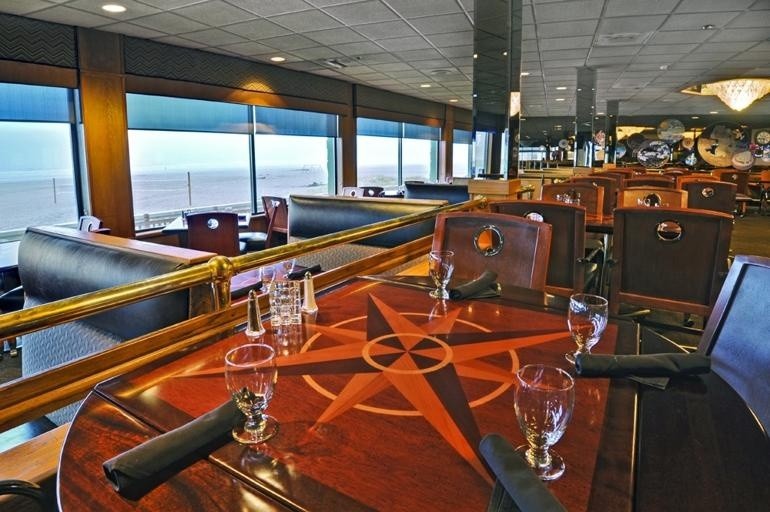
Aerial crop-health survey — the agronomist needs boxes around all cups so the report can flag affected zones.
[223,343,279,446]
[565,292,609,366]
[512,362,575,483]
[267,280,303,327]
[428,250,456,300]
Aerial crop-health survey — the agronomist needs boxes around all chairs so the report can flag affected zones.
[699,255,768,436]
[665,167,689,172]
[364,188,384,196]
[490,199,585,298]
[248,216,269,233]
[431,211,551,291]
[677,176,720,190]
[608,168,632,171]
[713,170,750,215]
[587,174,624,208]
[620,177,675,188]
[262,196,288,246]
[0,481,53,512]
[343,187,363,197]
[759,180,770,215]
[79,215,100,232]
[664,171,683,177]
[91,228,110,234]
[604,170,632,178]
[678,180,737,214]
[632,173,674,181]
[187,213,247,257]
[619,187,687,209]
[609,207,731,317]
[0,287,25,313]
[541,183,604,214]
[572,176,616,218]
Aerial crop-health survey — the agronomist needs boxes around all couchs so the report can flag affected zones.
[520,175,569,185]
[520,177,542,200]
[544,169,573,171]
[0,226,216,451]
[287,196,445,281]
[405,182,469,203]
[524,170,571,175]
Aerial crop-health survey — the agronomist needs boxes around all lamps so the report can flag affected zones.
[682,78,770,112]
